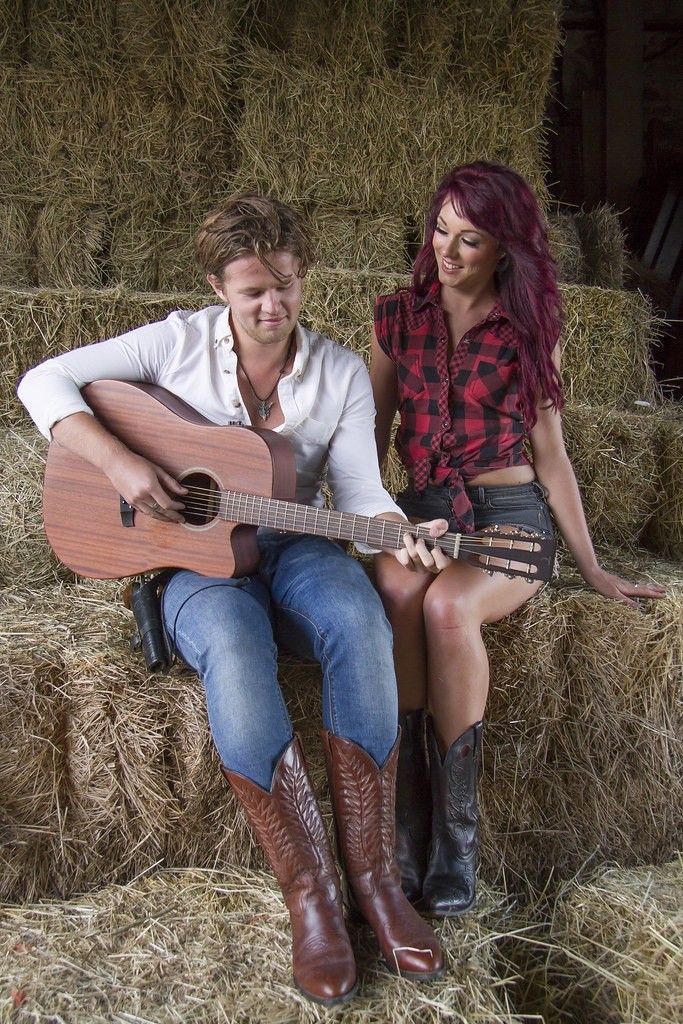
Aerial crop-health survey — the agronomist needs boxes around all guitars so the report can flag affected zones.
[43,377,559,587]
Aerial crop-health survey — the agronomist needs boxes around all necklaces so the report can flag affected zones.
[232,335,295,421]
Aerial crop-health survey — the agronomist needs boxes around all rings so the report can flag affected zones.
[634,583,639,588]
[151,502,160,510]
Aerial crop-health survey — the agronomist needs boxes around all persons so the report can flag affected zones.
[330,159,666,917]
[18,198,452,1006]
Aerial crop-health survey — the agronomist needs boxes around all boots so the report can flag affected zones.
[393,707,433,906]
[218,731,359,1006]
[423,709,484,916]
[321,721,447,981]
